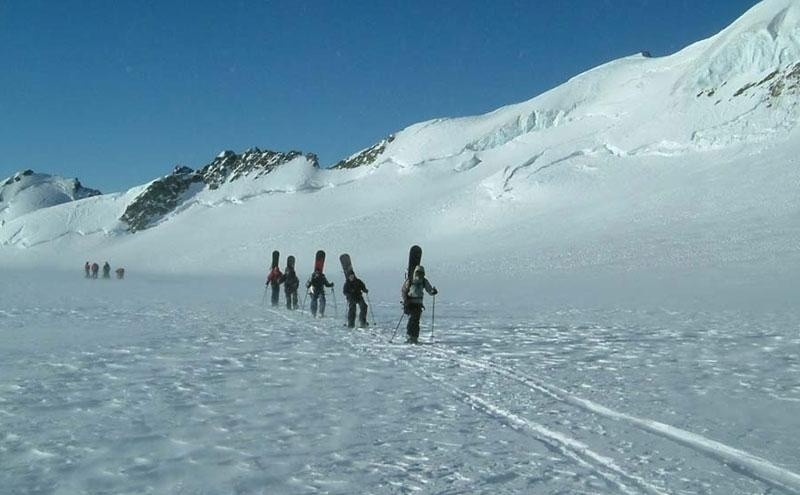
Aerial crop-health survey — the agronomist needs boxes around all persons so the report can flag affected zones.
[401,265,437,345]
[343,270,368,328]
[278,266,299,310]
[115,269,124,280]
[103,262,111,279]
[266,264,283,307]
[92,263,99,280]
[305,267,334,319]
[85,262,91,279]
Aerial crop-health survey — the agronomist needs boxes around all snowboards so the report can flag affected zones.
[408,244,421,279]
[272,251,279,268]
[287,256,295,269]
[339,253,353,279]
[315,251,326,273]
